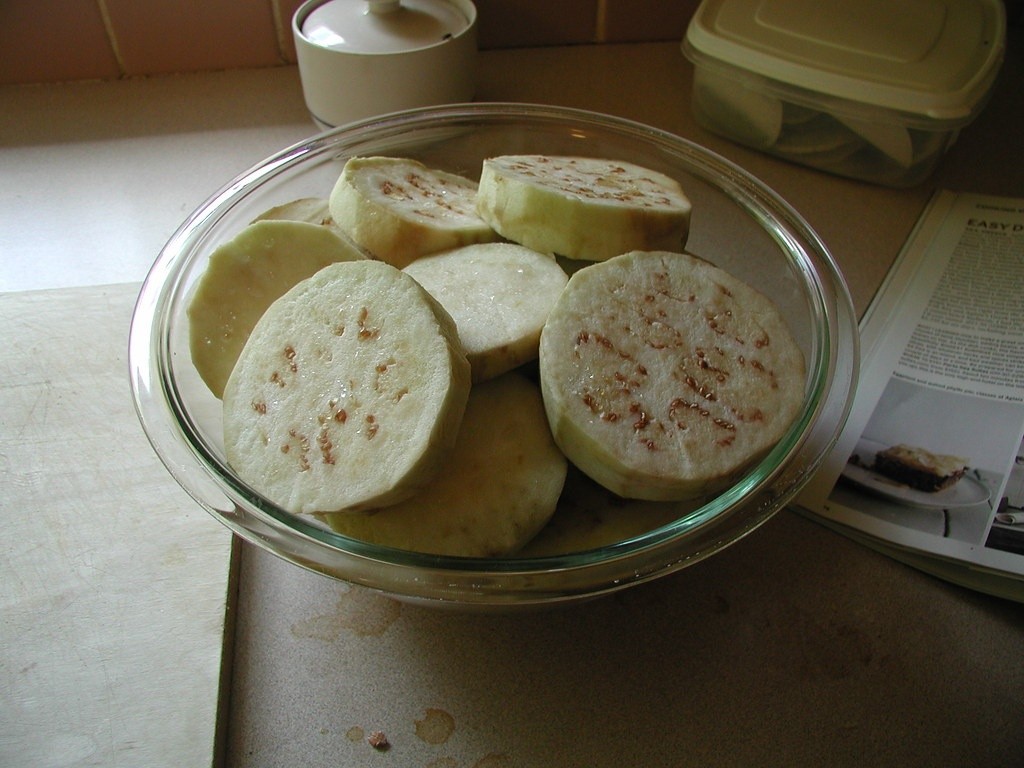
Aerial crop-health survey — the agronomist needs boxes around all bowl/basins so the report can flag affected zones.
[680,0,1008,189]
[127,105,864,610]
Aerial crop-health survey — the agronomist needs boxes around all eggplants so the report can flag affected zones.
[187,153,808,595]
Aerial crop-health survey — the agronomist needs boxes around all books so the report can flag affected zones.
[769,187,1024,602]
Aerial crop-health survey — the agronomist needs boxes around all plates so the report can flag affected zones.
[844,438,990,509]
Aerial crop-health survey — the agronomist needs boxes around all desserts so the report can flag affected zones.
[875,443,968,493]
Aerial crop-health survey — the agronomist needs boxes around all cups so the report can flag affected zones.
[292,0,485,131]
